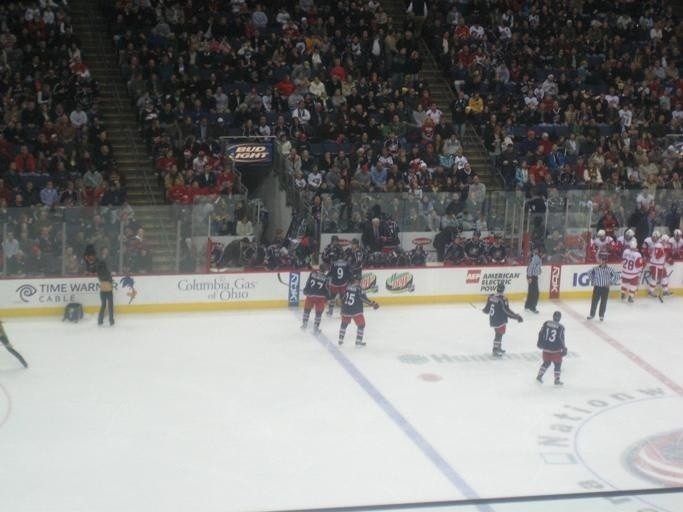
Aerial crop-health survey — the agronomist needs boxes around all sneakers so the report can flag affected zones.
[492,346,505,356]
[535,376,564,385]
[299,310,366,346]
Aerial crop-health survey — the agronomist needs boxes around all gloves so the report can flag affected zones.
[561,348,567,357]
[517,315,524,323]
[373,303,380,310]
[537,341,543,349]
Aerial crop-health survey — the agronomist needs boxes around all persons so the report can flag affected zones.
[587,258,619,320]
[302,238,378,346]
[0,1,682,279]
[523,247,546,313]
[620,242,673,302]
[0,325,28,368]
[536,311,567,384]
[483,283,523,358]
[99,261,114,326]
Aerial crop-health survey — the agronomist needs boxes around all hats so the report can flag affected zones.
[596,226,681,250]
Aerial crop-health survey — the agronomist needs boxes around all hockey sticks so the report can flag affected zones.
[277,272,304,291]
[335,299,374,308]
[470,303,485,312]
[658,269,674,304]
